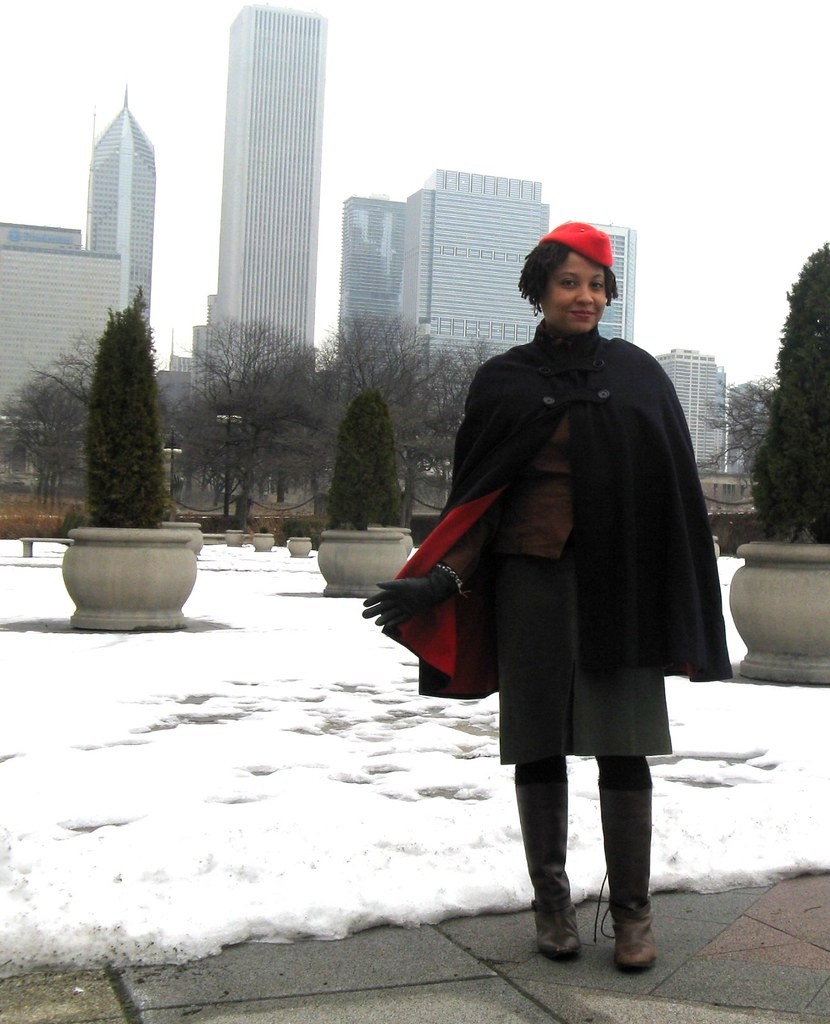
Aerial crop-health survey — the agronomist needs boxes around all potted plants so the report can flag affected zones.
[253,526,274,552]
[226,521,244,547]
[287,530,312,558]
[318,387,413,599]
[729,241,829,685]
[63,285,197,630]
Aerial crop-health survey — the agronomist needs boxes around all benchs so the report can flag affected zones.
[21,538,74,557]
[203,533,250,545]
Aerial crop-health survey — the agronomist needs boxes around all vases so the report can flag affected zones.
[161,522,204,555]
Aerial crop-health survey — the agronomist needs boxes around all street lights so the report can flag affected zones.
[444,458,450,503]
[162,448,184,499]
[216,414,242,515]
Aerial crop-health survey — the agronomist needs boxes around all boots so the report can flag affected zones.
[514,778,582,963]
[595,780,658,972]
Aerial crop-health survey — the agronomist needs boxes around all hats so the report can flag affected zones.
[539,223,612,268]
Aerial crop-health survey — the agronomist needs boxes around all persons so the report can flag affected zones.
[362,222,733,971]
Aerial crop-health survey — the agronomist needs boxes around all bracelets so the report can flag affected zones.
[437,562,465,597]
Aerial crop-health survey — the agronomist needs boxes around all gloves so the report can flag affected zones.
[361,563,462,631]
